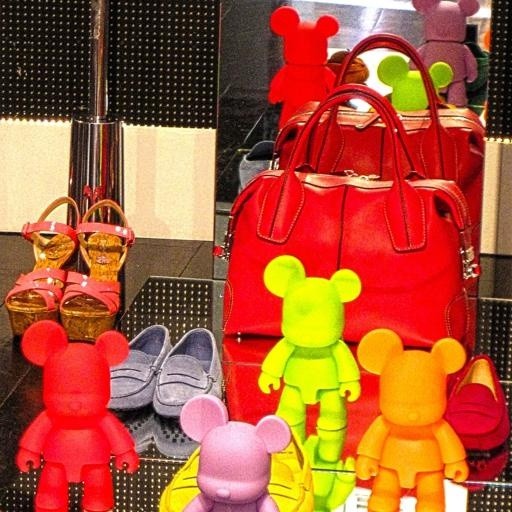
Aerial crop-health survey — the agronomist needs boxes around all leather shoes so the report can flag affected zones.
[152,328,223,418]
[105,324,172,409]
[463,41,491,92]
[443,355,511,451]
[236,140,280,190]
[159,443,201,512]
[327,48,369,83]
[266,415,315,512]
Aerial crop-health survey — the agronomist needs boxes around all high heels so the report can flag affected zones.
[59,199,135,342]
[4,197,81,336]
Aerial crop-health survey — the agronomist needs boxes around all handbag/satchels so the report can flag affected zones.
[213,84,482,353]
[272,32,488,267]
